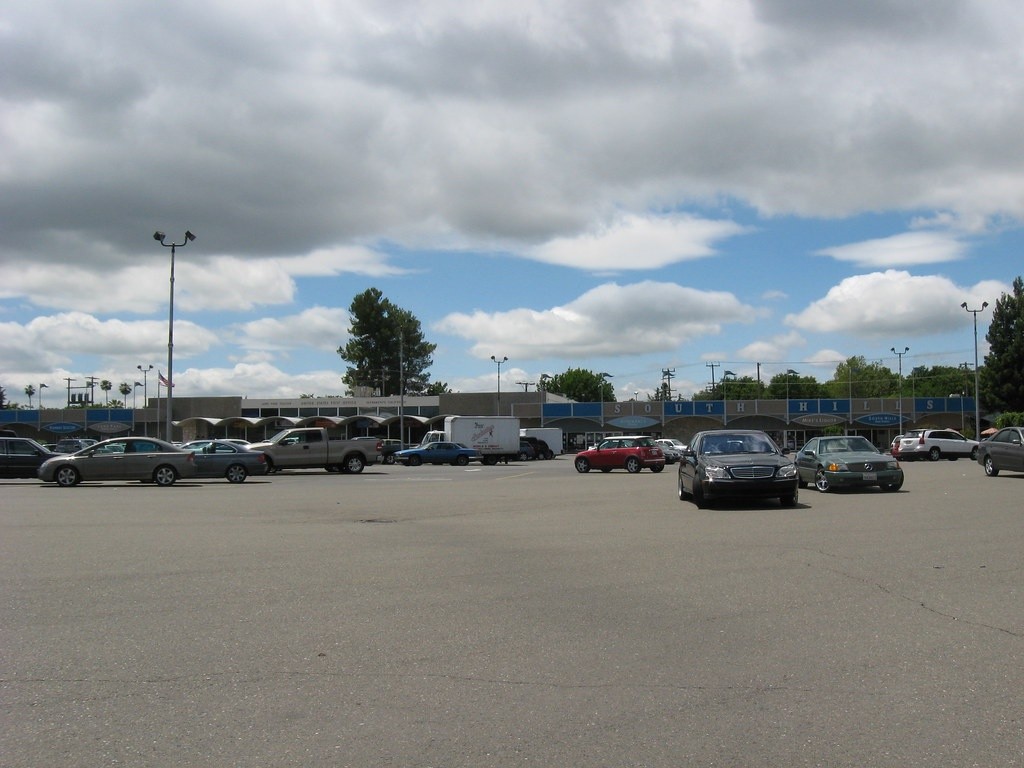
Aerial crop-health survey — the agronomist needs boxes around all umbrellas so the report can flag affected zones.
[981,428,998,436]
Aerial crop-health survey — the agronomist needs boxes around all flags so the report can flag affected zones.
[159,373,175,387]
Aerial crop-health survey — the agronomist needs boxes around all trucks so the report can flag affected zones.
[416,415,521,465]
[519,427,564,461]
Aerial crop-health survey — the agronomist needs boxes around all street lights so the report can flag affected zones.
[891,346,909,435]
[960,301,989,443]
[491,355,508,416]
[153,229,197,444]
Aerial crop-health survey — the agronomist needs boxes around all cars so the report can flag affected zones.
[588,437,690,465]
[677,428,800,510]
[520,436,550,462]
[0,428,273,490]
[179,439,270,484]
[36,435,198,488]
[977,426,1024,477]
[792,435,905,493]
[392,441,484,466]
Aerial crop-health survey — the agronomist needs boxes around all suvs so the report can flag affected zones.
[890,428,981,463]
[574,435,666,474]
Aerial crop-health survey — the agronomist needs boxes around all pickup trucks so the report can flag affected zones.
[241,425,410,476]
